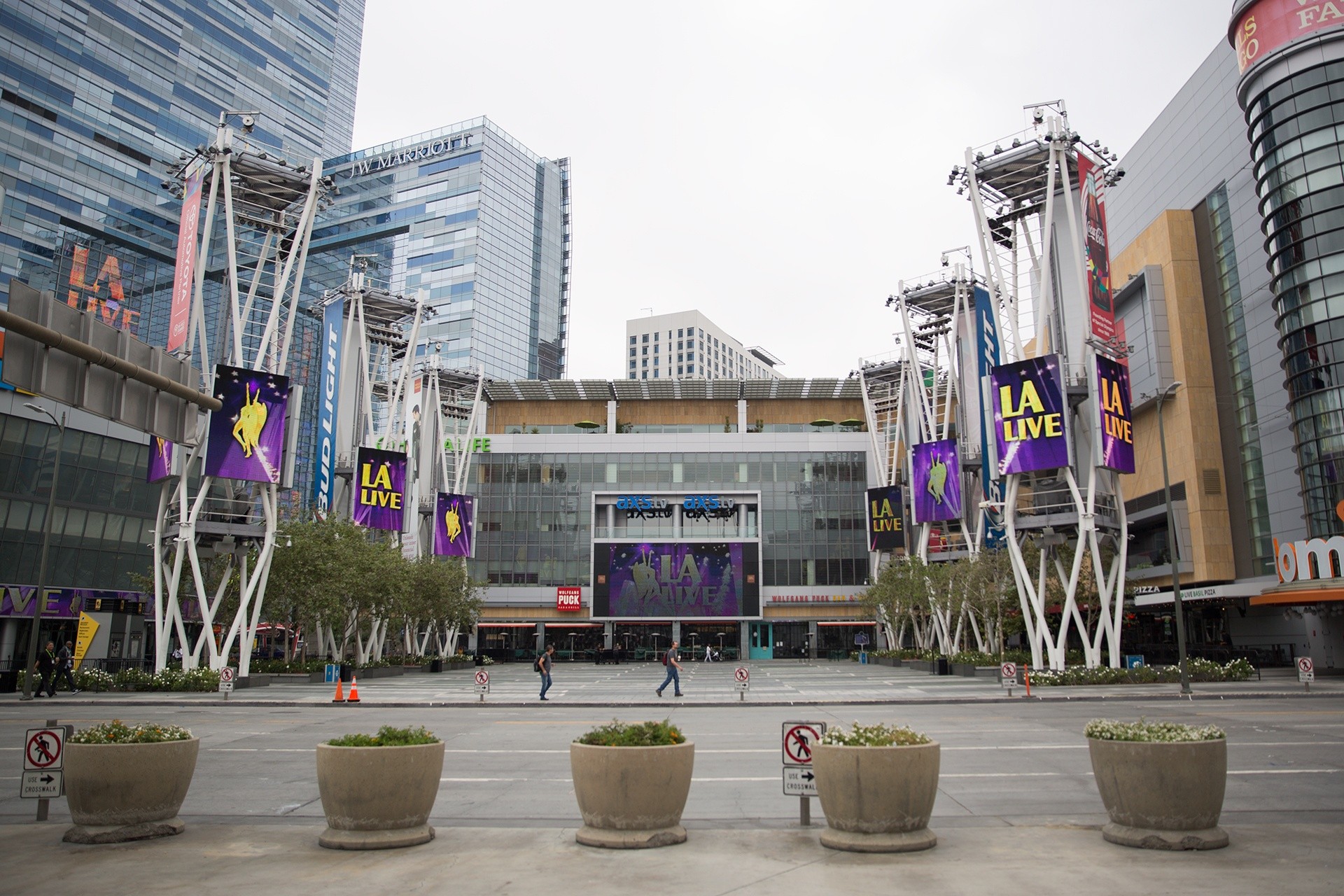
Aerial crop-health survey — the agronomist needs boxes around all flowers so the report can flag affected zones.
[580,721,686,748]
[1083,718,1227,745]
[66,717,194,745]
[816,719,931,748]
[326,725,444,749]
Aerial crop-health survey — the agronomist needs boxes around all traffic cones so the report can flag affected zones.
[347,676,361,703]
[332,678,346,702]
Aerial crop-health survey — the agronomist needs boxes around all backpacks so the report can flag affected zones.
[533,653,548,672]
[662,649,669,666]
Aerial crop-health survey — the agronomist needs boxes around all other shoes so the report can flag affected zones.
[539,694,549,700]
[49,691,55,697]
[675,693,683,696]
[34,695,44,698]
[656,689,662,697]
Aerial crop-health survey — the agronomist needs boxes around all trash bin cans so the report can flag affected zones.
[324,663,341,683]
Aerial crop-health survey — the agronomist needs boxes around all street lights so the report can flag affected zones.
[19,402,66,701]
[1159,380,1192,694]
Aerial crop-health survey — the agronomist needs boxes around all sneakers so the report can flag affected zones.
[72,688,82,695]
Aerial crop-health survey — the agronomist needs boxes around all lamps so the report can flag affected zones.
[299,163,305,172]
[243,115,256,135]
[235,215,299,236]
[453,368,479,378]
[315,174,341,219]
[279,156,287,166]
[259,149,267,160]
[397,293,403,299]
[441,403,470,420]
[410,295,415,303]
[339,287,347,294]
[421,305,439,323]
[160,142,232,214]
[359,286,365,294]
[367,326,405,348]
[307,290,331,312]
[384,289,389,297]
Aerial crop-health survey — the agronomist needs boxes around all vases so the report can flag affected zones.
[315,747,448,852]
[1087,734,1232,850]
[810,737,940,855]
[570,740,695,851]
[59,736,203,844]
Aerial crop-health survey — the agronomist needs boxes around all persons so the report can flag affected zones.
[539,645,554,700]
[33,641,57,698]
[703,643,713,662]
[1220,635,1226,647]
[51,640,82,695]
[593,644,602,664]
[655,641,684,697]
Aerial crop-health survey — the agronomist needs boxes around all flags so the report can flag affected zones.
[165,163,206,354]
[1077,151,1118,343]
